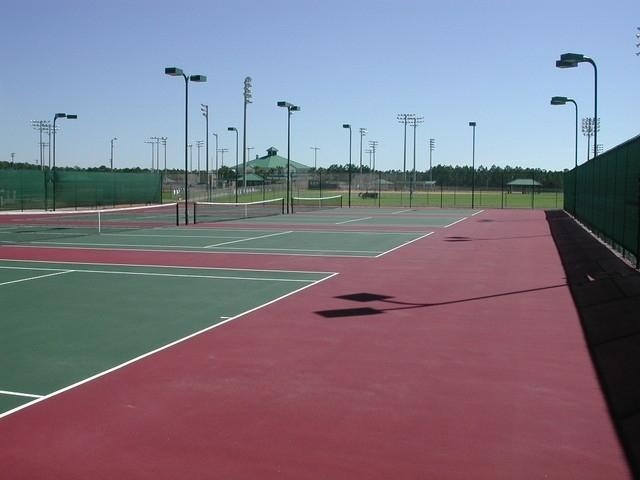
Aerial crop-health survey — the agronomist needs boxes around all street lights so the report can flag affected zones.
[555,51,599,160]
[549,95,606,168]
[634,25,640,57]
